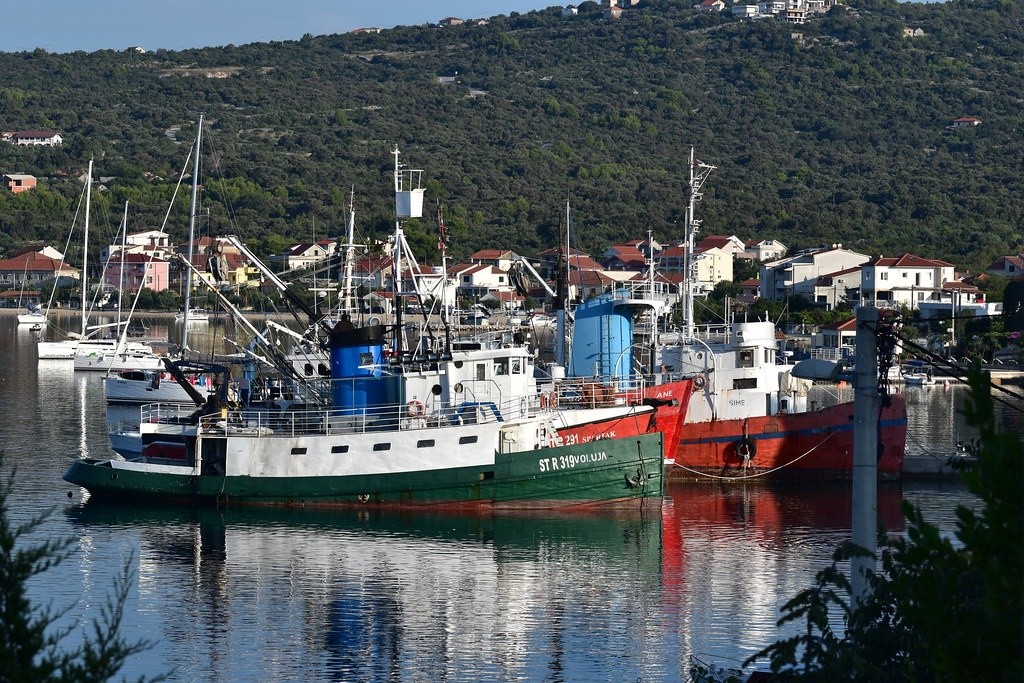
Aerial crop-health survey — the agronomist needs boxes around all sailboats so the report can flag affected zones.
[14,256,48,324]
[71,110,277,373]
[33,156,155,359]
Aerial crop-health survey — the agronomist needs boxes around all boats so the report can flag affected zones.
[173,295,211,321]
[214,139,938,477]
[29,323,42,332]
[62,208,669,514]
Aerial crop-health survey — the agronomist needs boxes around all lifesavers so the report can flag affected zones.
[735,439,757,460]
[549,392,556,407]
[539,392,547,410]
[406,400,425,418]
[667,322,675,332]
[693,375,707,391]
